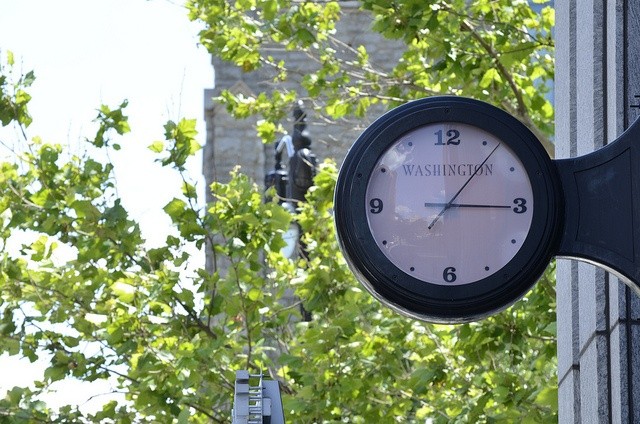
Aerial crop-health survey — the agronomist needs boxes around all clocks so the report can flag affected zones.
[333,97,558,324]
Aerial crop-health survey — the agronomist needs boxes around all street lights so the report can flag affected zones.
[264,103,311,326]
